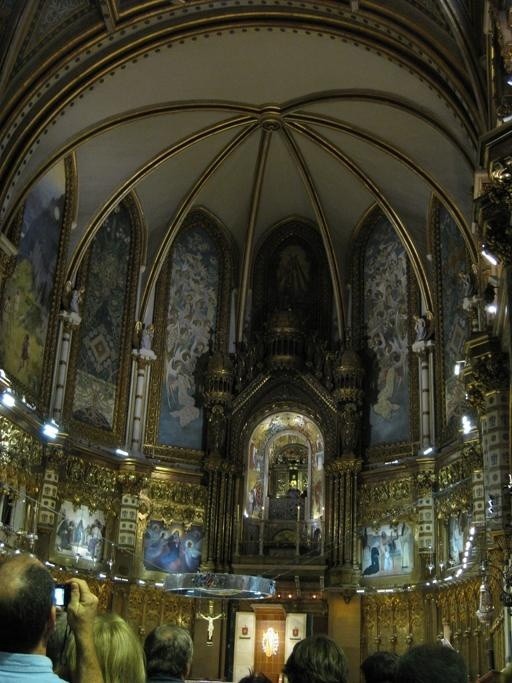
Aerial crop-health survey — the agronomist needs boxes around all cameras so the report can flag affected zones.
[53,584,71,607]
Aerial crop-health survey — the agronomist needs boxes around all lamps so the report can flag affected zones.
[476,577,512,624]
[480,560,512,614]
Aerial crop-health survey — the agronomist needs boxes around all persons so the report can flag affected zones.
[145,624,193,682]
[144,524,203,572]
[56,513,106,560]
[289,475,297,489]
[361,642,469,681]
[139,324,155,349]
[284,635,348,681]
[1,552,147,681]
[69,285,86,314]
[458,270,474,296]
[363,522,416,575]
[412,313,427,341]
